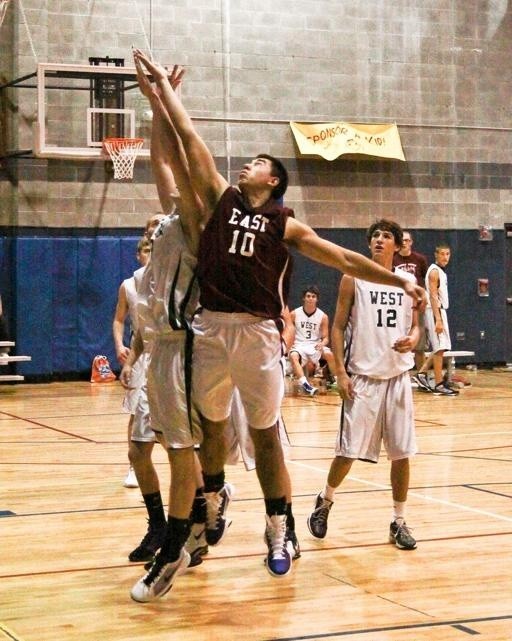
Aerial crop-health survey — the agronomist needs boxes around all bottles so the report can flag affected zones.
[451,357,456,370]
[288,373,338,395]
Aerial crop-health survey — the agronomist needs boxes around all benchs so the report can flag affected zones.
[411,350,476,388]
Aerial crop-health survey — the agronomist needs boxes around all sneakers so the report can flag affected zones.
[300,378,320,398]
[264,514,300,577]
[203,481,235,546]
[308,489,335,539]
[184,516,208,566]
[131,547,193,603]
[386,518,419,551]
[412,372,432,394]
[126,464,140,489]
[129,521,168,563]
[433,381,460,395]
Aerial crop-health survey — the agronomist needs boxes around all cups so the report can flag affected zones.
[444,374,464,390]
[466,365,476,371]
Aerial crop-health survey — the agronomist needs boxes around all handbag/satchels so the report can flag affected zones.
[90,355,116,382]
